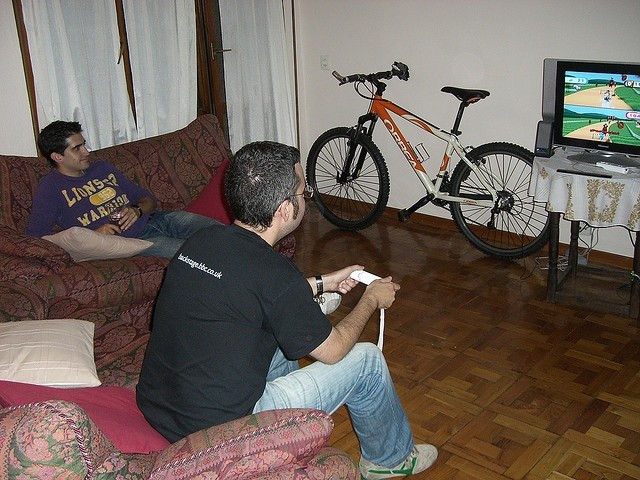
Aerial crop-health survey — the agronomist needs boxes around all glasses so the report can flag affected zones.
[279,185,313,211]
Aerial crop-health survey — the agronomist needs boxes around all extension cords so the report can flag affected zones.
[566,249,587,266]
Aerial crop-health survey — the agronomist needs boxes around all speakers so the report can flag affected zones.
[535,121,555,158]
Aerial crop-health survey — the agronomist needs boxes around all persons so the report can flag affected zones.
[25,120,228,260]
[135,142,439,466]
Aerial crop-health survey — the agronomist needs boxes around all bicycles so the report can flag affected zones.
[307,62,550,258]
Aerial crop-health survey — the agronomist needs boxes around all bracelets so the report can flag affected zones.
[314,273,324,303]
[129,202,142,214]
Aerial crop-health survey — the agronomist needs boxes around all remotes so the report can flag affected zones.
[596,160,629,175]
[349,270,382,286]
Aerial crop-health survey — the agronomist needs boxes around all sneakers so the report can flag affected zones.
[359,443,438,476]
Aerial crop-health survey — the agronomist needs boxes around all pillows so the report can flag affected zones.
[148,406,334,478]
[0,380,171,453]
[0,398,145,480]
[1,318,101,389]
[185,160,234,227]
[42,227,154,261]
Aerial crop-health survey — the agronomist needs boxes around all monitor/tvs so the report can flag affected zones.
[542,58,640,173]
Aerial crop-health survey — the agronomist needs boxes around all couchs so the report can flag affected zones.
[0,114,296,305]
[0,256,359,479]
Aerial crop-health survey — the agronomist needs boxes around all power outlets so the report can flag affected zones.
[578,221,590,235]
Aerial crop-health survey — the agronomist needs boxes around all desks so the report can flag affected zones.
[527,145,640,321]
